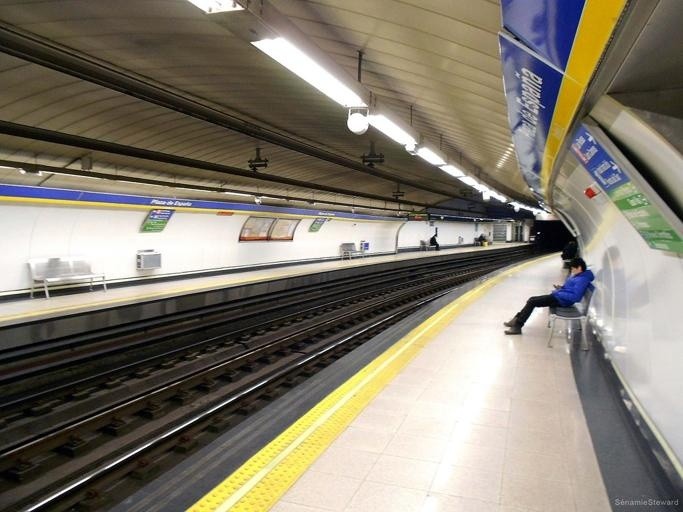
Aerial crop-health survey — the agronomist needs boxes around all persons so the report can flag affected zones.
[560,240,577,260]
[429,233,439,251]
[484,231,493,245]
[502,256,595,337]
[478,232,485,246]
[595,244,652,321]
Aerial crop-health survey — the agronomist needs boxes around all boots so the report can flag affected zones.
[505,326,521,335]
[504,317,522,327]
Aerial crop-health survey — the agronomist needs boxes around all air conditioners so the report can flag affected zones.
[136,252,162,270]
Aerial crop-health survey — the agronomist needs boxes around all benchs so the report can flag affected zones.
[28,253,108,300]
[420,240,436,251]
[547,282,598,351]
[563,247,580,268]
[474,238,482,246]
[341,243,365,260]
